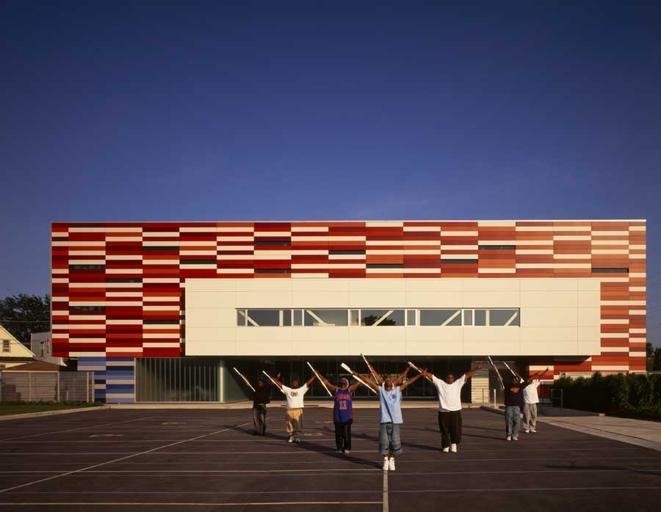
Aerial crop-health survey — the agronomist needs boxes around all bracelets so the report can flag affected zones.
[358,372,359,377]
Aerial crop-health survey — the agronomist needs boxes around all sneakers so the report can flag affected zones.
[382,457,396,471]
[443,444,458,453]
[336,449,349,455]
[507,436,519,441]
[288,436,302,443]
[524,429,536,433]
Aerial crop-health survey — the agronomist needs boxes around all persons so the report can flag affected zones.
[350,367,427,471]
[490,365,534,440]
[419,362,484,452]
[275,374,317,443]
[314,370,361,454]
[513,367,549,432]
[248,371,280,436]
